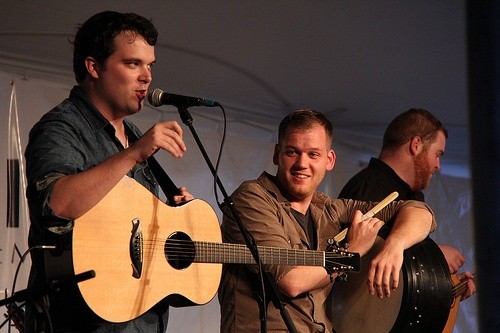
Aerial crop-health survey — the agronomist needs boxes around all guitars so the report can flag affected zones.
[442,273,478,333]
[56,174,361,324]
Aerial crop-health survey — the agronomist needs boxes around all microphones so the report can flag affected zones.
[147,88,220,108]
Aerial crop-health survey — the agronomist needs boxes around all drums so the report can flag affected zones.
[324,235,452,333]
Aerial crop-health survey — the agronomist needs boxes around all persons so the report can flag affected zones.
[218,109,437,333]
[337,109,477,299]
[24,11,194,333]
